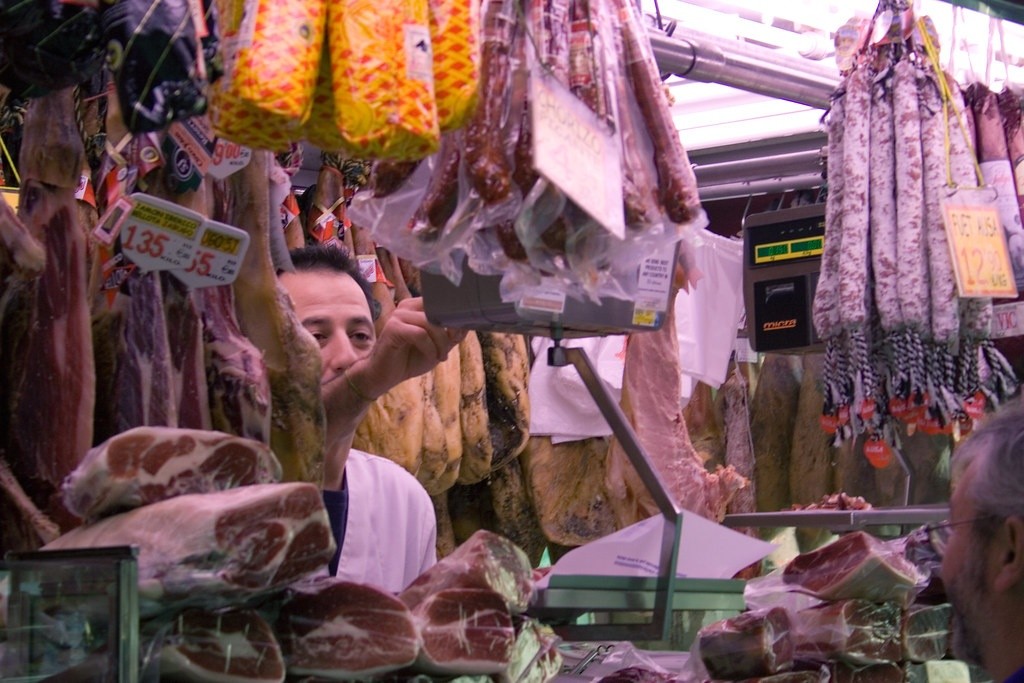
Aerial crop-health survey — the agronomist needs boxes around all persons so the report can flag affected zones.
[272,243,472,593]
[940,396,1024,683]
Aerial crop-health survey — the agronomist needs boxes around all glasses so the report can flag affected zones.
[925,514,990,558]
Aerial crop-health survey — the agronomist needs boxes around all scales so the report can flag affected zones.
[418,231,751,651]
[723,201,950,529]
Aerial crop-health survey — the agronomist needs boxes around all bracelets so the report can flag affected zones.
[344,369,378,403]
